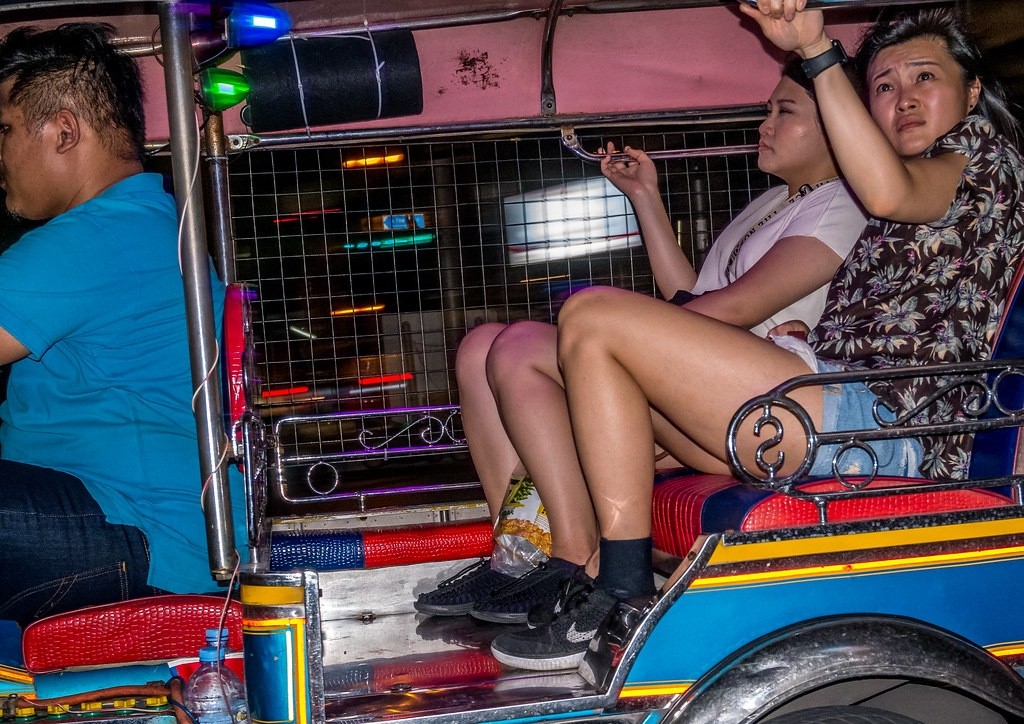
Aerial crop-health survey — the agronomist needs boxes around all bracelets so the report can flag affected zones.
[801,40,849,80]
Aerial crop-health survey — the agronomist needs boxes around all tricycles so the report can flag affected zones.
[0,2,1024,724]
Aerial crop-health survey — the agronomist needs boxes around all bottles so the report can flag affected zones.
[183,648,248,724]
[205,629,233,655]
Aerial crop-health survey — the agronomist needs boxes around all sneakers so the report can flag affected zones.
[491,576,659,670]
[471,555,587,623]
[527,565,600,629]
[413,557,551,615]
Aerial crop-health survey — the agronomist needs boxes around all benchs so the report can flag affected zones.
[644,252,1024,578]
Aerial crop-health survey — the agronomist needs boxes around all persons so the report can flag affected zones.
[0,20,251,634]
[414,0,1024,668]
